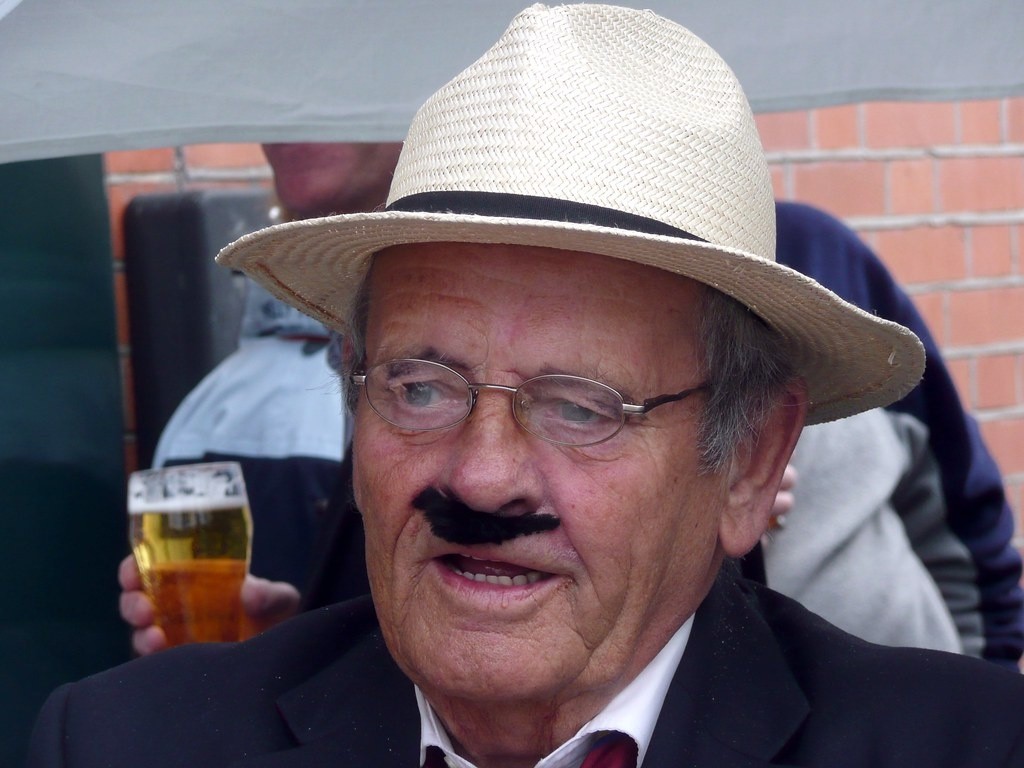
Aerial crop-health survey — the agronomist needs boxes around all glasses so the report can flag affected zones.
[348,351,712,446]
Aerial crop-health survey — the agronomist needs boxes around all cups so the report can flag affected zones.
[126,463,251,649]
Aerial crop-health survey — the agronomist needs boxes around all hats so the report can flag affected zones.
[215,5,928,427]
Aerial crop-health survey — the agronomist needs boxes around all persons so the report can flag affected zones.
[0,2,1024,768]
[763,200,1024,670]
[119,270,369,655]
